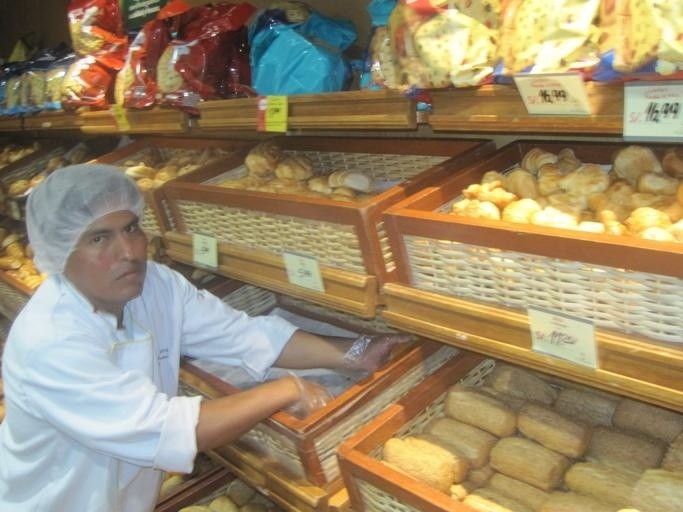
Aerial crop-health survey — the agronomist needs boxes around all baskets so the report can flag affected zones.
[0,139,109,222]
[0,269,45,323]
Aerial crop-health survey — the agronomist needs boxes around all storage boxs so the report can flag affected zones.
[0,134,66,191]
[0,239,34,318]
[153,468,279,511]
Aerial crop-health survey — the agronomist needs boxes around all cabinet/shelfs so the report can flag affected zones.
[1,66,683,512]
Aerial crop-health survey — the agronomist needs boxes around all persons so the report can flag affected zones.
[0,165,418,512]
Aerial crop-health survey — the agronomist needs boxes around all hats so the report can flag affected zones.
[25,163,145,274]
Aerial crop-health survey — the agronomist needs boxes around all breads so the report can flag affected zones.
[8,146,88,196]
[0,232,48,290]
[381,364,682,512]
[159,472,186,497]
[177,478,276,512]
[120,145,238,193]
[449,144,683,242]
[216,141,375,207]
[0,141,40,171]
